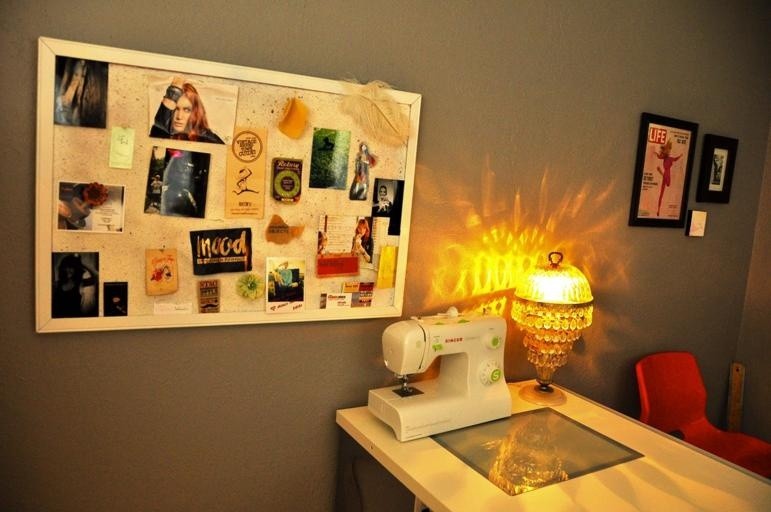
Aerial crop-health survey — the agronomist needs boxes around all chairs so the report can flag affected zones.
[634,351,771,479]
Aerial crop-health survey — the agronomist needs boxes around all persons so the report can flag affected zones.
[348,141,376,200]
[160,154,199,217]
[274,258,300,289]
[371,184,394,214]
[53,252,100,317]
[150,174,163,208]
[351,219,374,262]
[149,74,226,144]
[653,135,685,217]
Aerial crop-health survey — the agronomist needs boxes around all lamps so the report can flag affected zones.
[508,250,594,410]
[488,411,570,497]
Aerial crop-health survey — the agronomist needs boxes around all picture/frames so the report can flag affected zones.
[628,112,740,229]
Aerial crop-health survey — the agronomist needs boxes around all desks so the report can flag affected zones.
[334,377,770,512]
